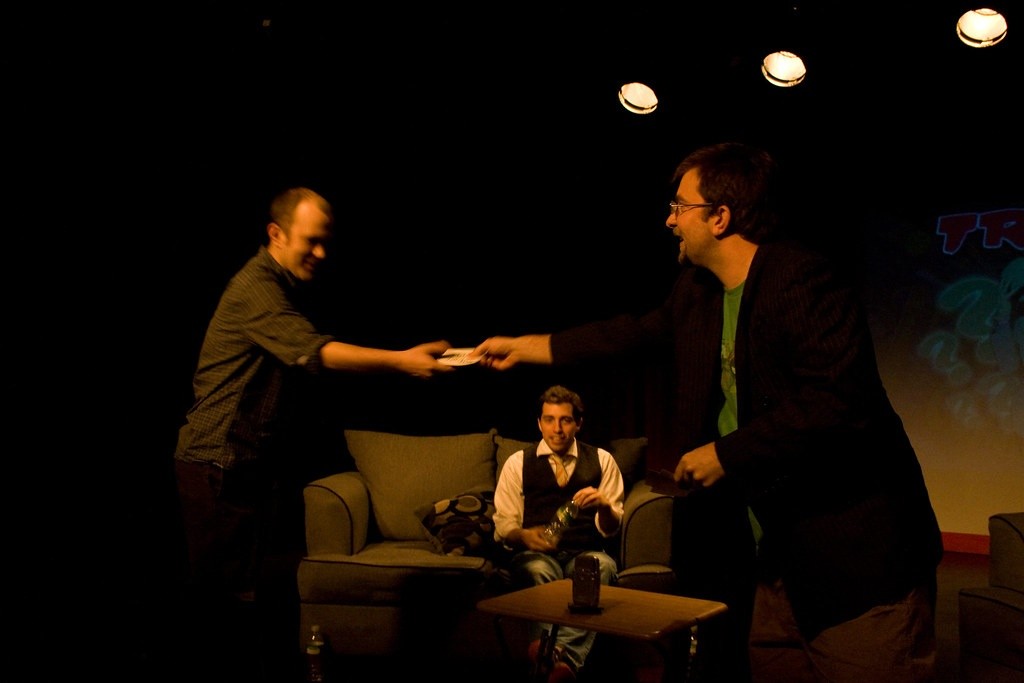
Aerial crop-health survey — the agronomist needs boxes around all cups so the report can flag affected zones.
[573,556,600,609]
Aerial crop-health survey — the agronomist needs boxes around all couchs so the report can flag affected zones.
[957,512,1024,683]
[291,428,688,654]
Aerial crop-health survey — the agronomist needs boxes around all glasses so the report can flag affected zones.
[669,201,713,214]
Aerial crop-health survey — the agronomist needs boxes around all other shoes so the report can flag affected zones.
[548,663,575,682]
[529,639,548,672]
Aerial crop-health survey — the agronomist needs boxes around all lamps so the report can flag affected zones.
[756,43,813,91]
[945,0,1009,50]
[613,60,674,121]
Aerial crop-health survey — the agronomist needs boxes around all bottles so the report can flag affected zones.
[542,500,578,548]
[306,625,325,683]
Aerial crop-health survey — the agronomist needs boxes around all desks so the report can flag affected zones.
[476,577,728,683]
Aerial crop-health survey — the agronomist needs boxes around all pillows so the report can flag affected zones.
[412,481,500,560]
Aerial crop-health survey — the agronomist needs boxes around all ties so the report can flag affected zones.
[552,455,568,487]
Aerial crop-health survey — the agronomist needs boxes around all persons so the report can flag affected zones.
[175,187,457,683]
[492,385,625,683]
[471,140,943,683]
